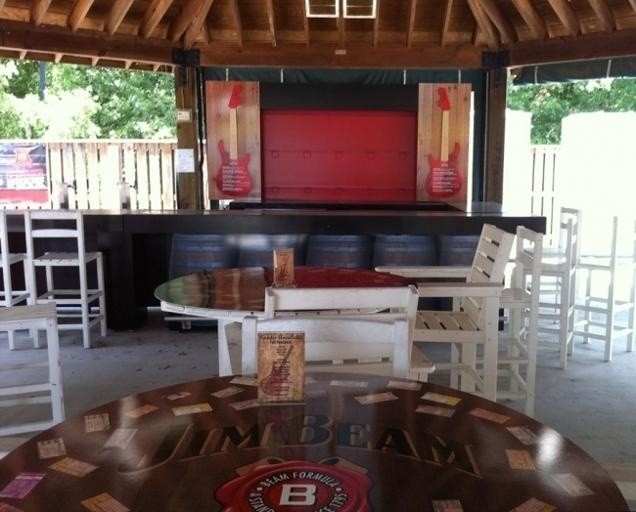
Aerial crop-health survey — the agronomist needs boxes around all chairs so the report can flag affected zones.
[377,222,514,401]
[544,206,581,324]
[510,216,580,366]
[0,207,107,442]
[451,226,543,418]
[238,284,433,379]
[580,215,633,363]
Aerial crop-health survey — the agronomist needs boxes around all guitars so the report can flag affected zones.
[215,84,253,197]
[259,344,294,396]
[426,87,462,197]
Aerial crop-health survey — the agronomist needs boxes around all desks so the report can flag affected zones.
[155,268,421,377]
[0,375,631,509]
